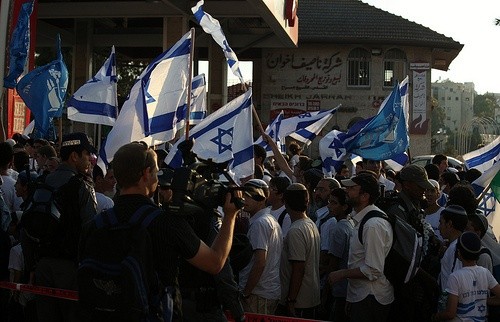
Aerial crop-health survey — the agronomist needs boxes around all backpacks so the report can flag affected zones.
[358,210,424,291]
[59,204,170,322]
[21,172,91,246]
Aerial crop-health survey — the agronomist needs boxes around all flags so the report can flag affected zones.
[2,0,34,90]
[190,0,243,81]
[253,74,410,180]
[462,133,500,193]
[97,27,208,164]
[16,33,69,139]
[66,45,118,128]
[162,86,256,183]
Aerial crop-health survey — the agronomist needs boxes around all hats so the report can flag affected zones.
[18,170,41,189]
[400,164,435,190]
[476,213,488,232]
[428,178,440,190]
[445,204,467,216]
[286,183,307,190]
[60,132,98,154]
[459,232,482,254]
[341,174,378,189]
[51,156,61,165]
[246,178,269,189]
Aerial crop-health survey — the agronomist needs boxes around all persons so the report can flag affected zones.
[0,133,500,322]
[326,173,397,322]
[76,142,244,321]
[431,231,500,322]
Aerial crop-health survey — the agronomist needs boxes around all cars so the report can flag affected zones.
[409,155,463,170]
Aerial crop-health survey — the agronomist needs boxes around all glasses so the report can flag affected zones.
[313,187,330,193]
[327,198,339,205]
